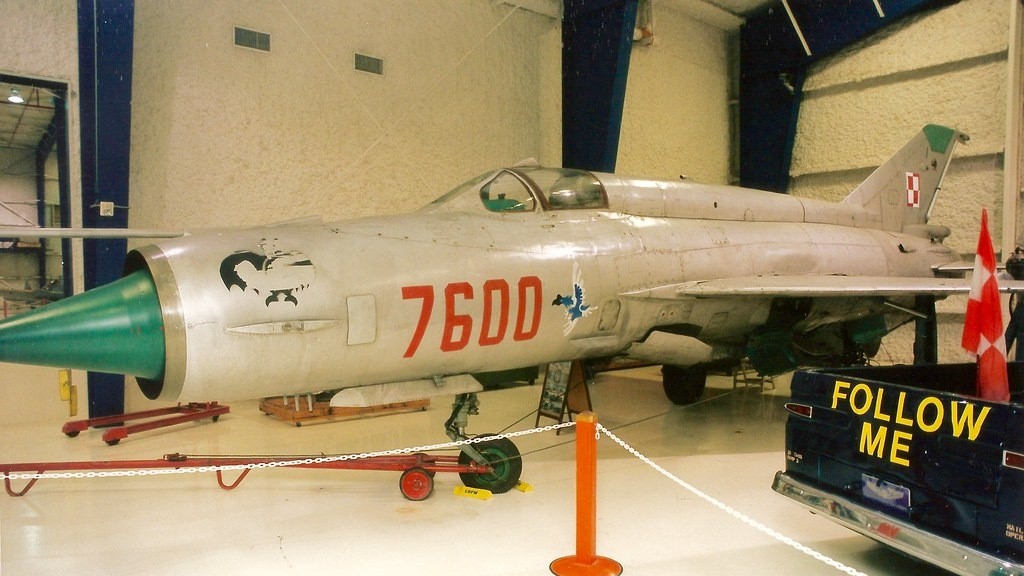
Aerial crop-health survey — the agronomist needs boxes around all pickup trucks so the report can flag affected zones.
[771,360,1024,576]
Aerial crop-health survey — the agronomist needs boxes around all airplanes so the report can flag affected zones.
[0,124,1024,493]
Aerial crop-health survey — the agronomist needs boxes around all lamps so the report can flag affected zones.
[8,88,24,103]
